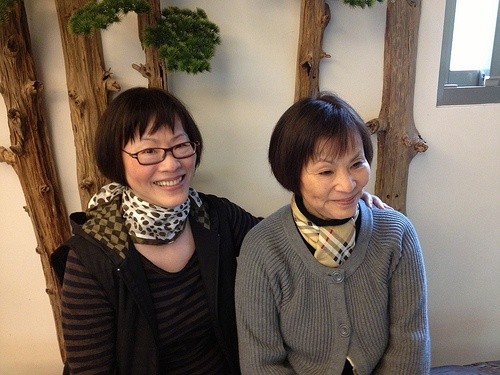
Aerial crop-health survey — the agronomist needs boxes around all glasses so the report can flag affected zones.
[122,140,199,166]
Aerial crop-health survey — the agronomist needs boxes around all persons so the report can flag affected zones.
[48,88,394,375]
[235,91,432,375]
